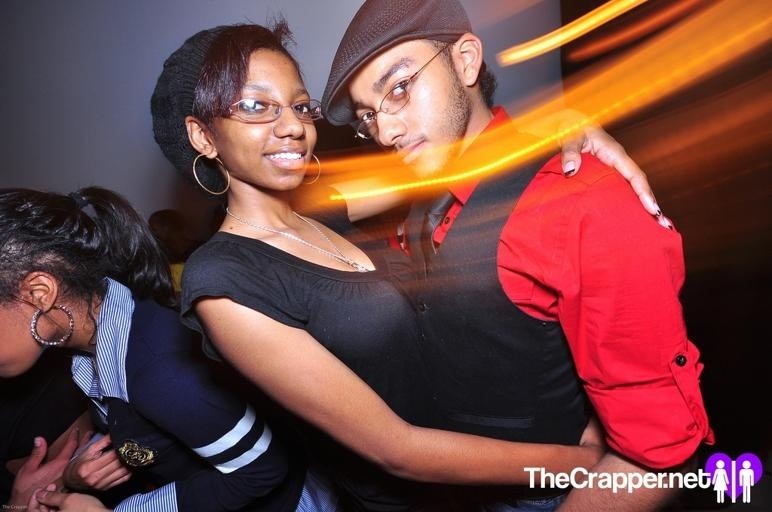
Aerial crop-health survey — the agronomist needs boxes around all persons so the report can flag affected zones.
[319,0,720,512]
[0,410,94,512]
[143,12,680,512]
[0,186,345,512]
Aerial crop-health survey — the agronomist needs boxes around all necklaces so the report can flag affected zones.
[223,206,376,274]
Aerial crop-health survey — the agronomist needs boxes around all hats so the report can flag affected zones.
[321,0,474,128]
[148,24,228,197]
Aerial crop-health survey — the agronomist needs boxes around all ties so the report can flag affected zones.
[420,190,457,274]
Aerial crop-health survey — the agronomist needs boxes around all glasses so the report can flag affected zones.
[216,95,325,125]
[352,40,453,141]
